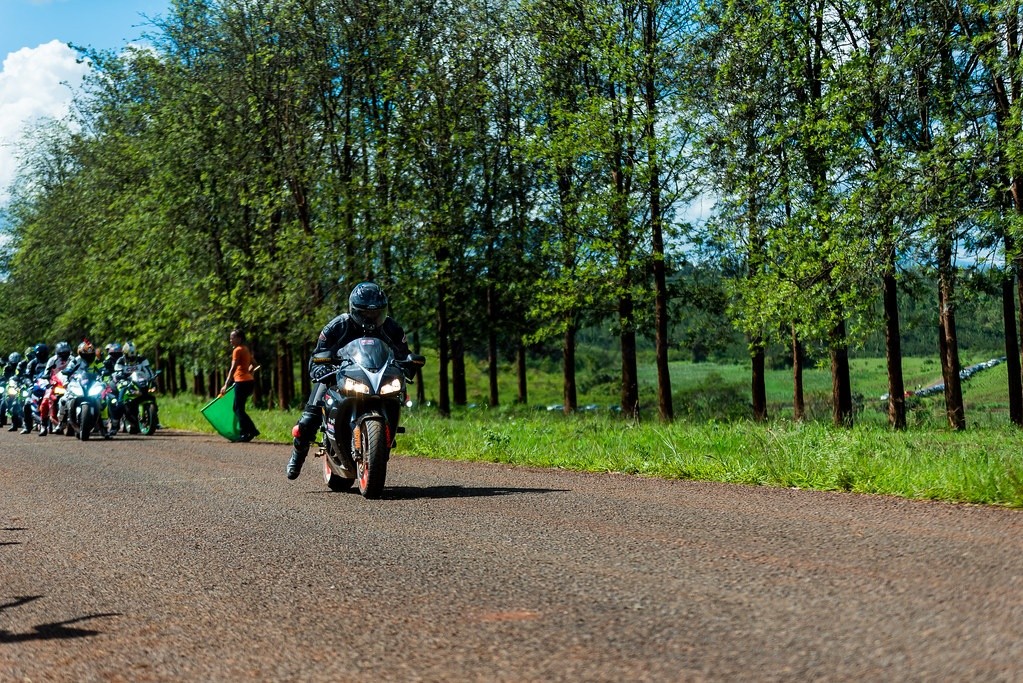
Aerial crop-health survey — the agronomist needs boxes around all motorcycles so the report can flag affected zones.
[116,370,161,434]
[0,376,73,437]
[63,367,109,441]
[315,336,426,497]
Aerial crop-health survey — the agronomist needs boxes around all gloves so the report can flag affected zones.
[315,365,336,384]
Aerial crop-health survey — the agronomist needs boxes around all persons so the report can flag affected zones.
[220,329,260,443]
[286,282,425,480]
[0,340,161,439]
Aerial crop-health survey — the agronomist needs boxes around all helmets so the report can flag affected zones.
[348,282,388,330]
[8,337,139,362]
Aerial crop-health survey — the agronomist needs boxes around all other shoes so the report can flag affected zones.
[238,431,260,443]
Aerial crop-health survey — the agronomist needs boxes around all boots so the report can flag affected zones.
[286,437,309,480]
[0,409,160,439]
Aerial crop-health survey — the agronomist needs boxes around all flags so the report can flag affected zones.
[202,386,241,441]
